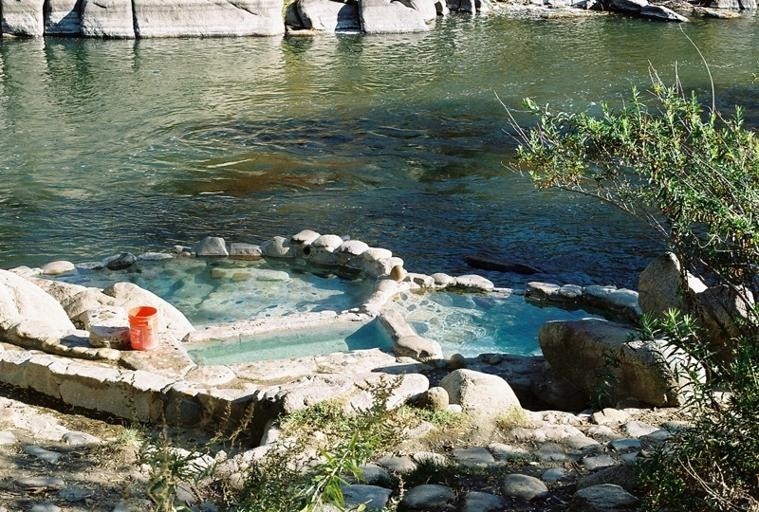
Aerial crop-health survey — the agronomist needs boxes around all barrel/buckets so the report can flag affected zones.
[128,306,159,350]
